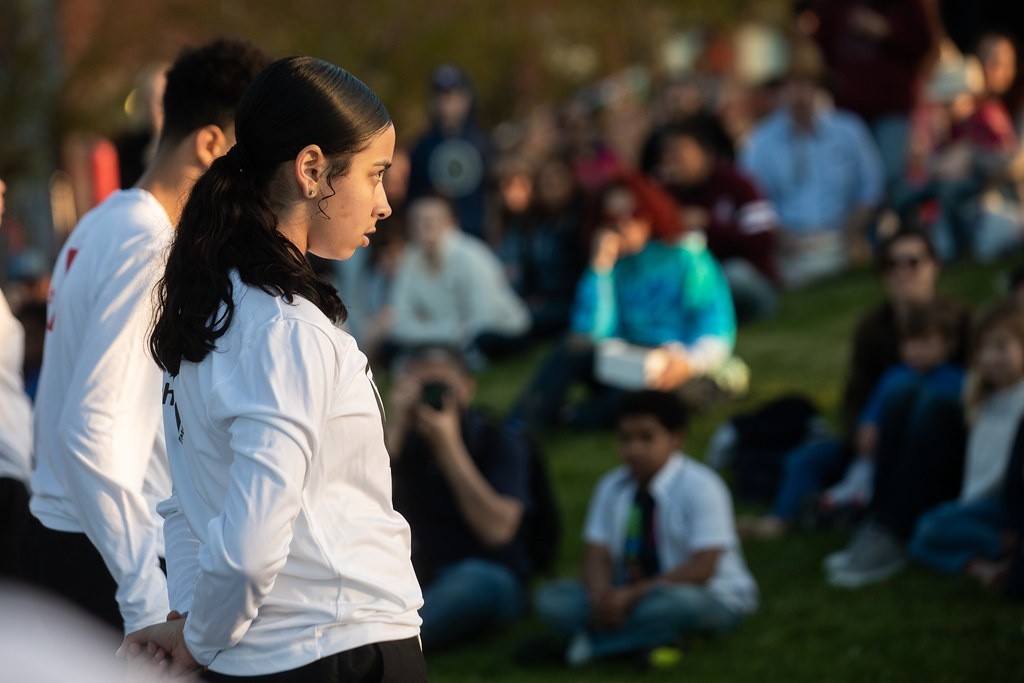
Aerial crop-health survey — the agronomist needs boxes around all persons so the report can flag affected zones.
[92,0,1024,351]
[380,339,562,646]
[119,54,427,683]
[534,176,751,433]
[521,386,762,669]
[30,37,263,640]
[734,227,1024,603]
[0,172,39,590]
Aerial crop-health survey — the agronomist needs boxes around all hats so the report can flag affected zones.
[429,59,471,90]
[598,179,646,220]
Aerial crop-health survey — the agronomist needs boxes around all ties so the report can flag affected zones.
[619,471,661,582]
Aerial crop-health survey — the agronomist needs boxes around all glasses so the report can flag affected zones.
[880,251,935,273]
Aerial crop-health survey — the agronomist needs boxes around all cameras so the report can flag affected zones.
[415,377,451,411]
